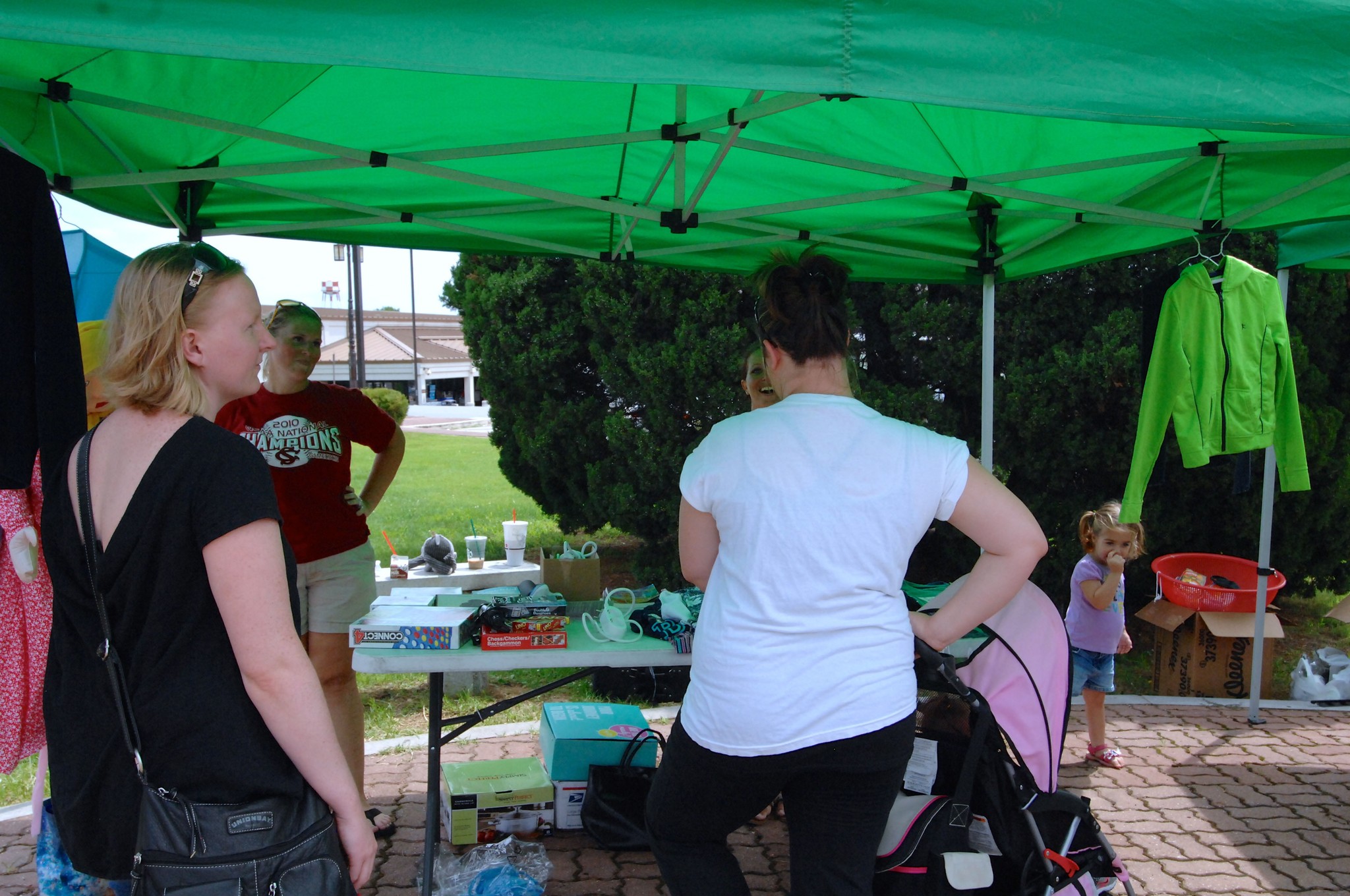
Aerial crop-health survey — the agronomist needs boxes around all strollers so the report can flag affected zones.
[871,571,1136,896]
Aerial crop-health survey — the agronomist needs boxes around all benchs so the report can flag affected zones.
[374,559,540,698]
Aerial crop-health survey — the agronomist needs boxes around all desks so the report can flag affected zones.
[352,600,991,896]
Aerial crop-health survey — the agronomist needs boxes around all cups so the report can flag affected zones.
[501,520,528,567]
[465,536,487,569]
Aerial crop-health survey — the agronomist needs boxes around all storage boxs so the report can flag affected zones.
[349,586,570,650]
[1135,600,1285,701]
[440,702,659,845]
[539,547,600,605]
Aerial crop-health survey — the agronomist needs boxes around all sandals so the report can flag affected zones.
[1086,742,1123,767]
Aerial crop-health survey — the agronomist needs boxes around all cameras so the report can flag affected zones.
[477,602,511,633]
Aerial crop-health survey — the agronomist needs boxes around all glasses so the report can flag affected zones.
[181,239,227,317]
[269,298,322,338]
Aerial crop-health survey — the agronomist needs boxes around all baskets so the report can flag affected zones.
[1152,553,1287,612]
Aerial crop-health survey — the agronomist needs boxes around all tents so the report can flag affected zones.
[0,0,1350,715]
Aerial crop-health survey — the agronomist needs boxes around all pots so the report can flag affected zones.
[488,807,543,836]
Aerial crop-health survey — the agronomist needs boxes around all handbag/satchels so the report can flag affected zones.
[130,775,359,896]
[580,728,667,850]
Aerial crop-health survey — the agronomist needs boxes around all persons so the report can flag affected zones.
[913,558,1070,792]
[40,243,376,896]
[742,343,781,411]
[214,299,405,835]
[1063,499,1142,767]
[639,245,1050,896]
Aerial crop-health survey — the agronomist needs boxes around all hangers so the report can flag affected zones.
[1178,218,1233,287]
